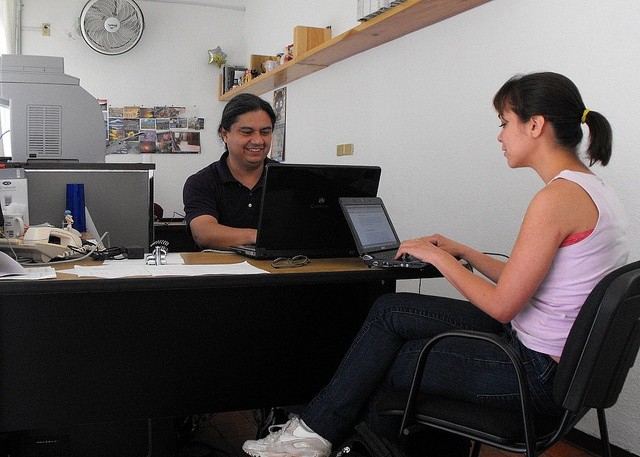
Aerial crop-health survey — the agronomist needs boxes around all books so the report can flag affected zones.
[357,0,403,22]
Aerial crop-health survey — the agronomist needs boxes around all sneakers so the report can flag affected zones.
[242,416,333,457]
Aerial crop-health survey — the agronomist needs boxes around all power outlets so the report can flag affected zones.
[41,22,50,37]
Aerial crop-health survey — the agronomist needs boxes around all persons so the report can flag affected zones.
[241,71,631,456]
[181,92,280,252]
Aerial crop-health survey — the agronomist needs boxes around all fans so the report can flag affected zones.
[77,0,144,56]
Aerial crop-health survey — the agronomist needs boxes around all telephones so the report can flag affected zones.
[0,226,84,263]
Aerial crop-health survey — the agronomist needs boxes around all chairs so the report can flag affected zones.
[378,260,640,456]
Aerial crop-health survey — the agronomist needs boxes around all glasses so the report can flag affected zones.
[271,255,311,268]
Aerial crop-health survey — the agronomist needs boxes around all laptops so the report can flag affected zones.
[20,168,154,249]
[338,195,436,272]
[229,162,381,260]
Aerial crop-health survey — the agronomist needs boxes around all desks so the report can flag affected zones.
[0,247,469,457]
[154,219,184,229]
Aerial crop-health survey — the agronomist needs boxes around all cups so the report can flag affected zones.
[3,215,24,238]
